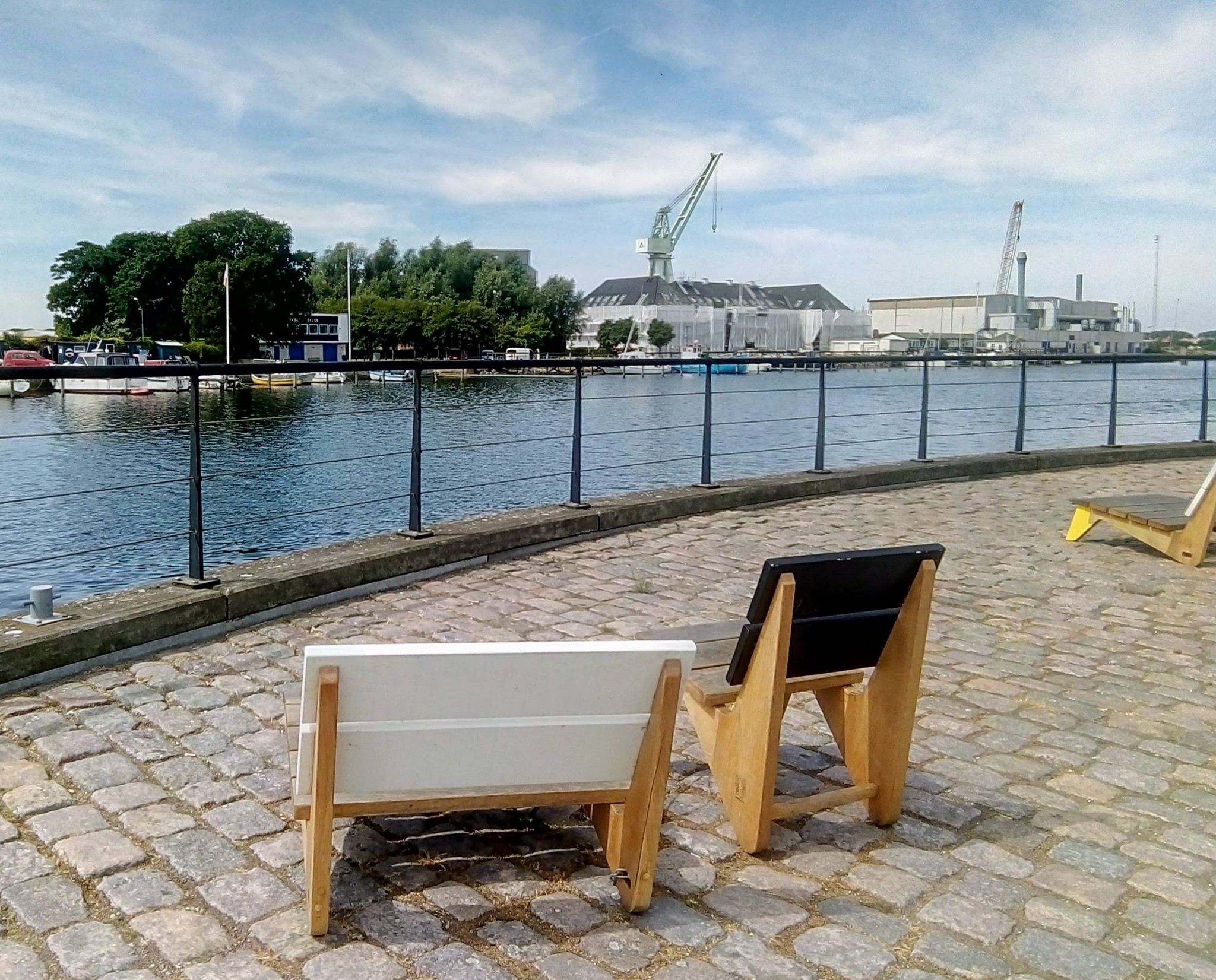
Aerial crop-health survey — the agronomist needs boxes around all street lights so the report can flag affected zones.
[134,297,144,341]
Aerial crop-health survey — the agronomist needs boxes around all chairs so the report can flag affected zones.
[282,640,698,940]
[1065,462,1216,566]
[635,543,946,854]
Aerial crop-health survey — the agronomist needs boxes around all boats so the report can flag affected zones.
[0,379,30,397]
[49,334,188,394]
[310,371,346,384]
[597,352,773,374]
[369,370,413,382]
[900,351,1082,367]
[251,372,316,385]
[143,359,239,389]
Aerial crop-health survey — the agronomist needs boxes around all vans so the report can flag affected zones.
[505,348,540,360]
[481,350,494,360]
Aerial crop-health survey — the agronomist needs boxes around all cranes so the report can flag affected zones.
[636,153,724,284]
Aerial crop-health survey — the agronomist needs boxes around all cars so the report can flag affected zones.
[3,351,56,366]
[448,349,468,360]
